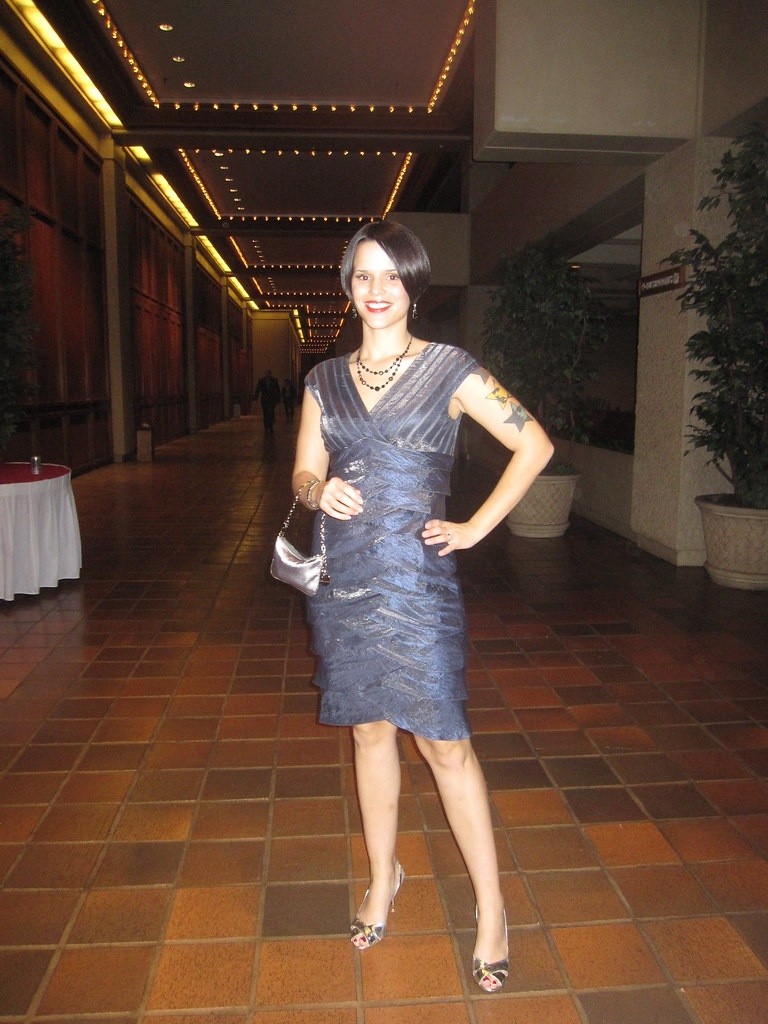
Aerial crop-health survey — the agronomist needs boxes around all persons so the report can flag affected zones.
[255,369,281,433]
[292,221,554,990]
[280,372,296,421]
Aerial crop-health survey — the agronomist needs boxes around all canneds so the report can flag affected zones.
[30,455,42,475]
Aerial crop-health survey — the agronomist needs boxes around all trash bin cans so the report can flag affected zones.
[135,427,157,465]
[231,400,241,420]
[249,401,257,416]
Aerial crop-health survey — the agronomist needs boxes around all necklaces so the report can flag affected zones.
[356,335,412,391]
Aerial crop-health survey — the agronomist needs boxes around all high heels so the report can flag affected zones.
[472,903,509,991]
[349,864,406,949]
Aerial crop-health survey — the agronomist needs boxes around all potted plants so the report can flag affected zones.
[482,231,611,538]
[660,119,768,591]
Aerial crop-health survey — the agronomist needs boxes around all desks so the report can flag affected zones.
[0,461,82,603]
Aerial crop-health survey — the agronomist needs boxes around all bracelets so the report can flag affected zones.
[308,483,319,507]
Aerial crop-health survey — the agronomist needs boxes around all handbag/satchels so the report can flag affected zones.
[268,477,330,598]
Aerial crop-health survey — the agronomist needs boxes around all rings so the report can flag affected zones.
[448,534,451,541]
